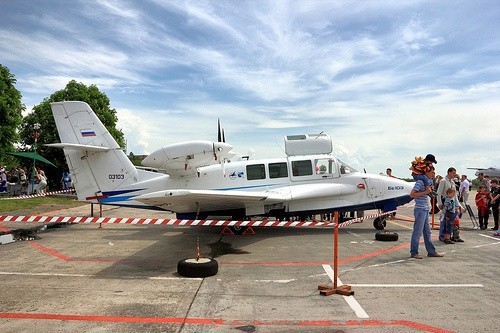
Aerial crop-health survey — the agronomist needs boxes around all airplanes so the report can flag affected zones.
[467,166,500,181]
[41,100,416,234]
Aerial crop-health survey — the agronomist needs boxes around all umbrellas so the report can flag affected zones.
[4,151,58,194]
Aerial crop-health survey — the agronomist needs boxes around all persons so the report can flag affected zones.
[386,167,397,216]
[410,154,439,198]
[433,167,500,244]
[410,166,444,259]
[0,162,48,195]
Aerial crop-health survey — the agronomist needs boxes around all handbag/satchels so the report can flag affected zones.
[460,196,463,202]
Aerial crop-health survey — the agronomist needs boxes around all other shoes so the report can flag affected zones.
[411,253,422,259]
[493,232,500,236]
[429,211,432,214]
[451,237,464,242]
[439,235,444,241]
[484,225,487,229]
[480,226,484,230]
[444,238,454,244]
[428,252,444,257]
[434,210,440,214]
[432,191,438,196]
[491,227,497,230]
[426,192,434,198]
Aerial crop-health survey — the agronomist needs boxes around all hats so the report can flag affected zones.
[425,154,437,164]
